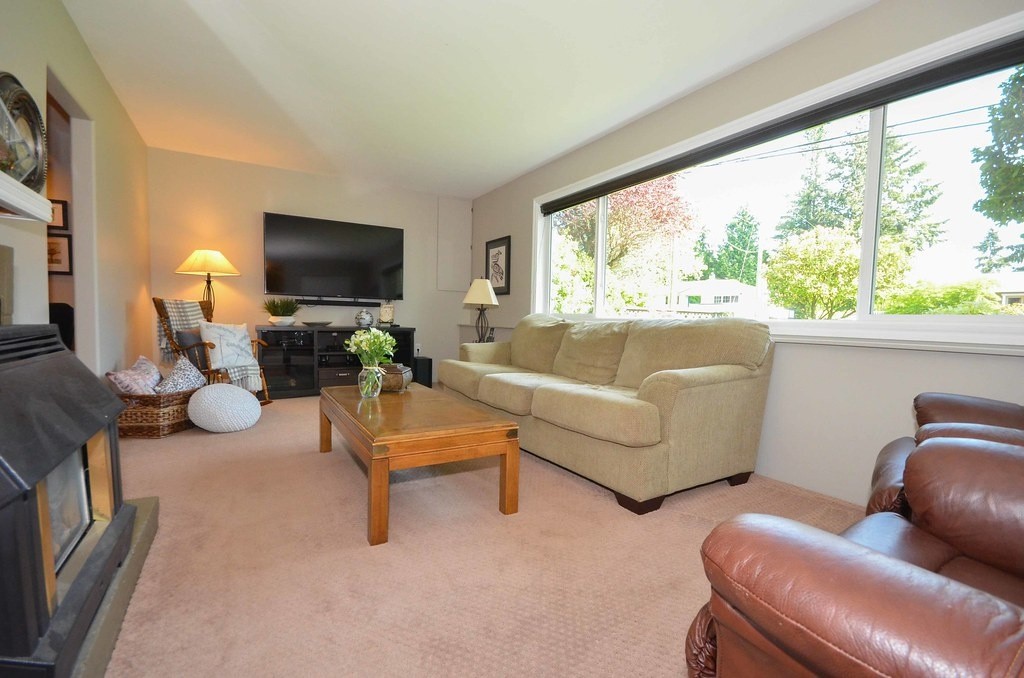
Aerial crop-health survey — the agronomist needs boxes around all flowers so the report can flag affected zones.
[345,327,397,395]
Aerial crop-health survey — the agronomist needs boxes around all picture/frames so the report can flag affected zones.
[48,233,73,275]
[485,234,511,294]
[47,198,68,230]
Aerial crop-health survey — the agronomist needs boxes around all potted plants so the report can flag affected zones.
[262,297,302,326]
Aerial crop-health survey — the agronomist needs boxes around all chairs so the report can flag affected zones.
[153,297,272,405]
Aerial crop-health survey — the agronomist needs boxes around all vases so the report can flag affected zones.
[357,366,383,398]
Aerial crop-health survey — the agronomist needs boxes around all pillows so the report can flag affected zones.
[155,354,206,393]
[105,354,163,394]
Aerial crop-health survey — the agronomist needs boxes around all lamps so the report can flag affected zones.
[462,278,499,343]
[174,248,241,310]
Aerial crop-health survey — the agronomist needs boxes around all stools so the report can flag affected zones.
[187,383,263,433]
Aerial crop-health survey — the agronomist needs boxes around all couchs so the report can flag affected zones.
[687,437,1024,678]
[437,312,776,513]
[865,392,1024,516]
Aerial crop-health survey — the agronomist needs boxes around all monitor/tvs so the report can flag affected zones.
[262,211,404,300]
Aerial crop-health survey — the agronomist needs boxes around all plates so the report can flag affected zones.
[303,321,332,326]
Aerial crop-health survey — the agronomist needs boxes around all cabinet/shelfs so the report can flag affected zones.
[256,327,417,397]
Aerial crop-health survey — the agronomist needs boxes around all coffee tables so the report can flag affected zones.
[319,382,518,546]
